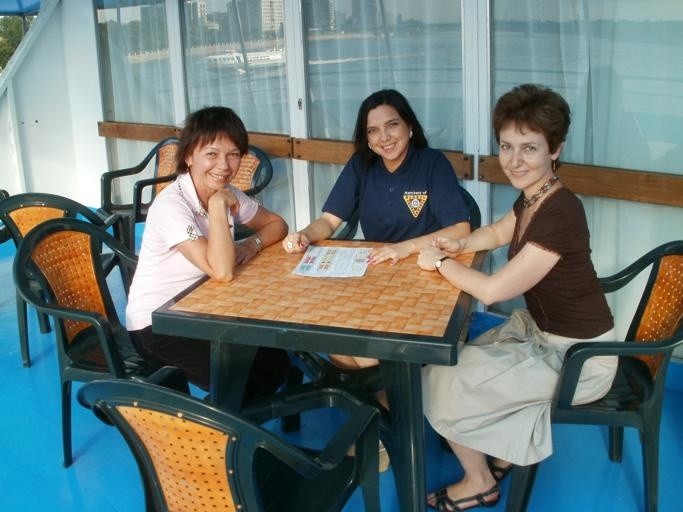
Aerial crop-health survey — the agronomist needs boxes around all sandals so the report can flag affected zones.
[427,484,500,512]
[489,461,512,481]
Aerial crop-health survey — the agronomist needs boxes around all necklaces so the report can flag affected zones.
[523,174,559,209]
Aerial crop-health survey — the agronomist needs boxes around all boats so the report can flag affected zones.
[202,46,285,73]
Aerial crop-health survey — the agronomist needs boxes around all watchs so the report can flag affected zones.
[433,256,451,274]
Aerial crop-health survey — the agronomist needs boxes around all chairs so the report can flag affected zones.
[504,238,683,512]
[73,376,383,512]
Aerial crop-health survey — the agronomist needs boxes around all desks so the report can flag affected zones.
[155,223,492,509]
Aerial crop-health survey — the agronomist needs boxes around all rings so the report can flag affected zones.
[286,240,293,249]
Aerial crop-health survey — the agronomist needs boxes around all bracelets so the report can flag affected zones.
[249,234,265,252]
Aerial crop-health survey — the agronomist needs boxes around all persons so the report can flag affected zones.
[415,82,621,512]
[123,105,292,406]
[281,87,474,476]
